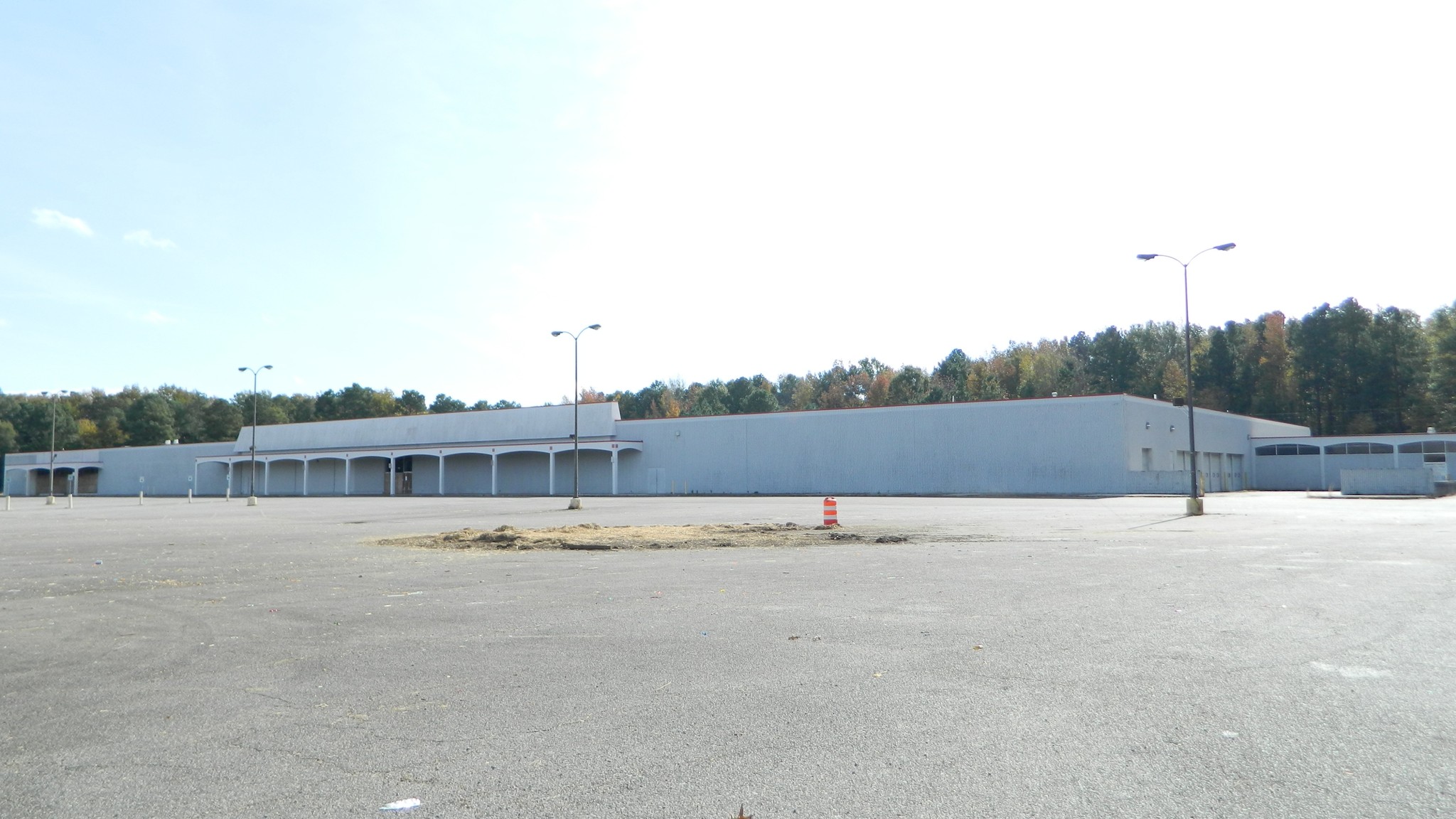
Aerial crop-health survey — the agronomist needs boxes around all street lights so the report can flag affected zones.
[238,365,274,497]
[41,390,68,496]
[1135,241,1236,498]
[551,324,602,498]
